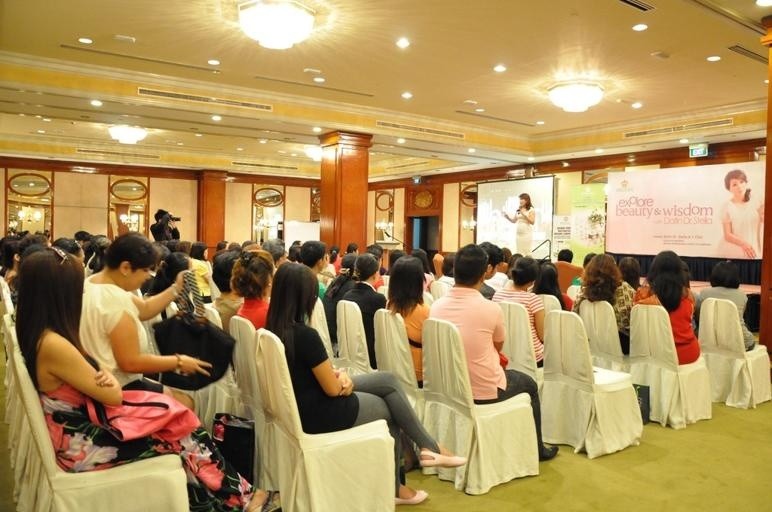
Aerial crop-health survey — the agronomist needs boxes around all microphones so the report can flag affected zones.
[385,231,390,238]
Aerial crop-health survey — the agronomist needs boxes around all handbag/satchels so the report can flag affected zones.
[633,382,651,426]
[86,390,202,445]
[152,312,236,391]
[211,411,256,486]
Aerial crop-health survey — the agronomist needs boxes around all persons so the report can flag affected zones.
[697,262,754,351]
[718,169,766,259]
[150,210,179,243]
[429,241,700,462]
[1,233,468,511]
[501,193,536,257]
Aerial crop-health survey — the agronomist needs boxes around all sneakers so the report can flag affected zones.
[248,489,283,511]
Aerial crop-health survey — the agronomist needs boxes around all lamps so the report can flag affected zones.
[236,0,318,49]
[547,80,605,113]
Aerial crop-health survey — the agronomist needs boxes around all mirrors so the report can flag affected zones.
[251,184,287,245]
[5,168,53,247]
[374,188,395,243]
[460,179,477,250]
[108,174,149,243]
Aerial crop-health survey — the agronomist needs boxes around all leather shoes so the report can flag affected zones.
[395,490,430,505]
[418,451,468,468]
[539,446,558,460]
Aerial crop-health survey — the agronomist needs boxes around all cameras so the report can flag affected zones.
[162,214,182,226]
